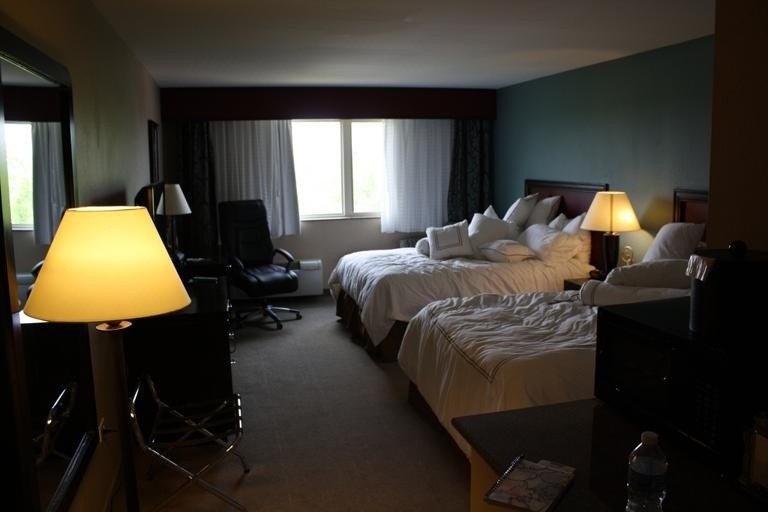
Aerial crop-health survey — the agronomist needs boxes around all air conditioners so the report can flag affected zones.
[229,258,324,301]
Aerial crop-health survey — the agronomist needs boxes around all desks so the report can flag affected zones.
[452,398,766,509]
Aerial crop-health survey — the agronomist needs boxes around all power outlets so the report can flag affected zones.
[98,418,106,443]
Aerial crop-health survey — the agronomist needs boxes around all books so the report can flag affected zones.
[485,447,576,512]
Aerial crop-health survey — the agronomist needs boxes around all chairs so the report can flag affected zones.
[214,199,302,329]
[130,382,249,511]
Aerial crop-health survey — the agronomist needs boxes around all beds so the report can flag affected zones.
[328,181,605,354]
[396,191,706,468]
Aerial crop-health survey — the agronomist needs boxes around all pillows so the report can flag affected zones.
[581,273,694,313]
[606,256,699,293]
[413,194,580,265]
[634,220,702,262]
[420,191,704,309]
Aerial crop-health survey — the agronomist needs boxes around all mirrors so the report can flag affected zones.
[0,28,98,511]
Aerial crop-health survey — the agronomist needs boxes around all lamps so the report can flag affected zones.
[156,183,192,269]
[23,205,193,333]
[581,188,640,270]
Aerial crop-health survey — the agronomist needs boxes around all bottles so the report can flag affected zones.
[623,431,672,512]
